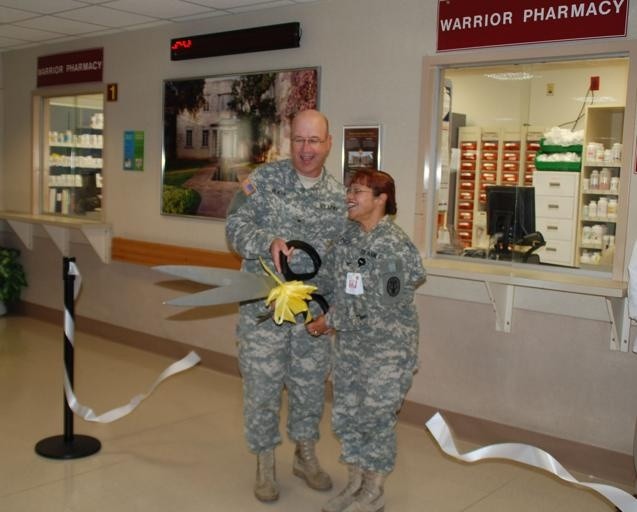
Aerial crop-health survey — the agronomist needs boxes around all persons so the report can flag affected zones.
[225,108,351,502]
[267,166,427,511]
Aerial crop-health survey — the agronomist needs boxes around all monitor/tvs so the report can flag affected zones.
[485,186,535,253]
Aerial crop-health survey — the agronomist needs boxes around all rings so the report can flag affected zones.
[314,331,319,335]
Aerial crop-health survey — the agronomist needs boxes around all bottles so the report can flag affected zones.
[50,111,104,186]
[581,142,621,264]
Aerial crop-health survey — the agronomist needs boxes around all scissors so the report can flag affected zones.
[149,239,330,326]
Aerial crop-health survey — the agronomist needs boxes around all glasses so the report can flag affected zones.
[345,187,376,196]
[289,133,328,147]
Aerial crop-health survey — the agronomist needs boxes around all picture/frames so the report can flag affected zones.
[160,65,322,224]
[342,124,382,186]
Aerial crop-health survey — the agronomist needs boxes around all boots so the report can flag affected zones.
[320,463,388,511]
[255,446,279,501]
[292,438,332,490]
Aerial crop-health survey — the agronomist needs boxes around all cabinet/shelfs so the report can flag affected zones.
[454,104,624,273]
